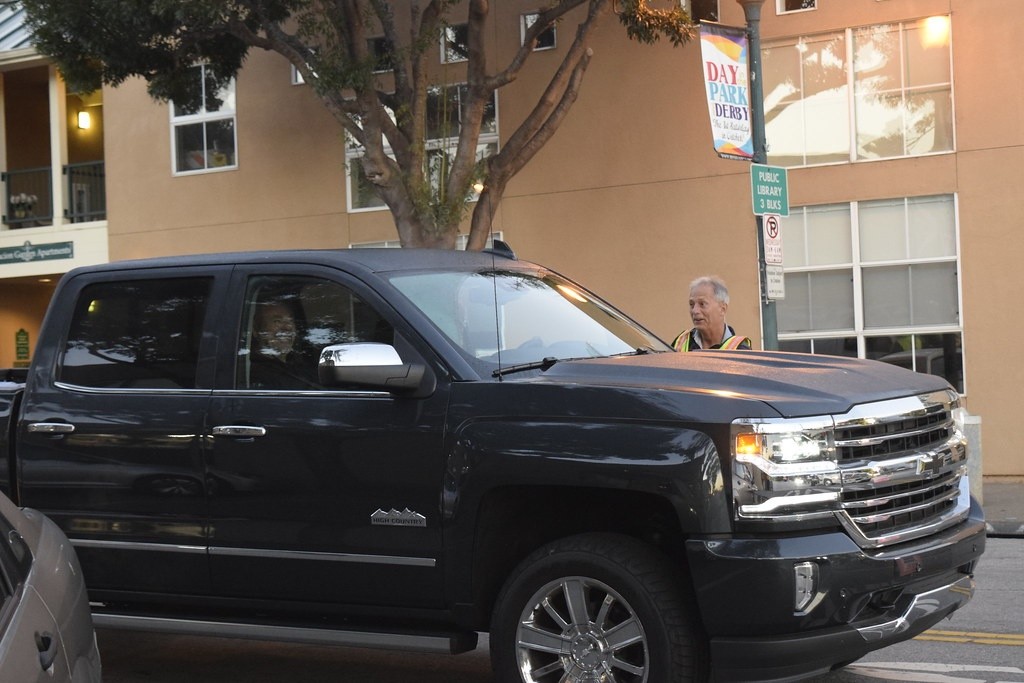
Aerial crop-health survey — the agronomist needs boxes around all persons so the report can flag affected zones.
[239,294,322,393]
[667,274,753,352]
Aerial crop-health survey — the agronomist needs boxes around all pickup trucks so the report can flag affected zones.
[0,245,988,683]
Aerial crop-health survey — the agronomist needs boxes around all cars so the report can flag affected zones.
[0,493,101,682]
